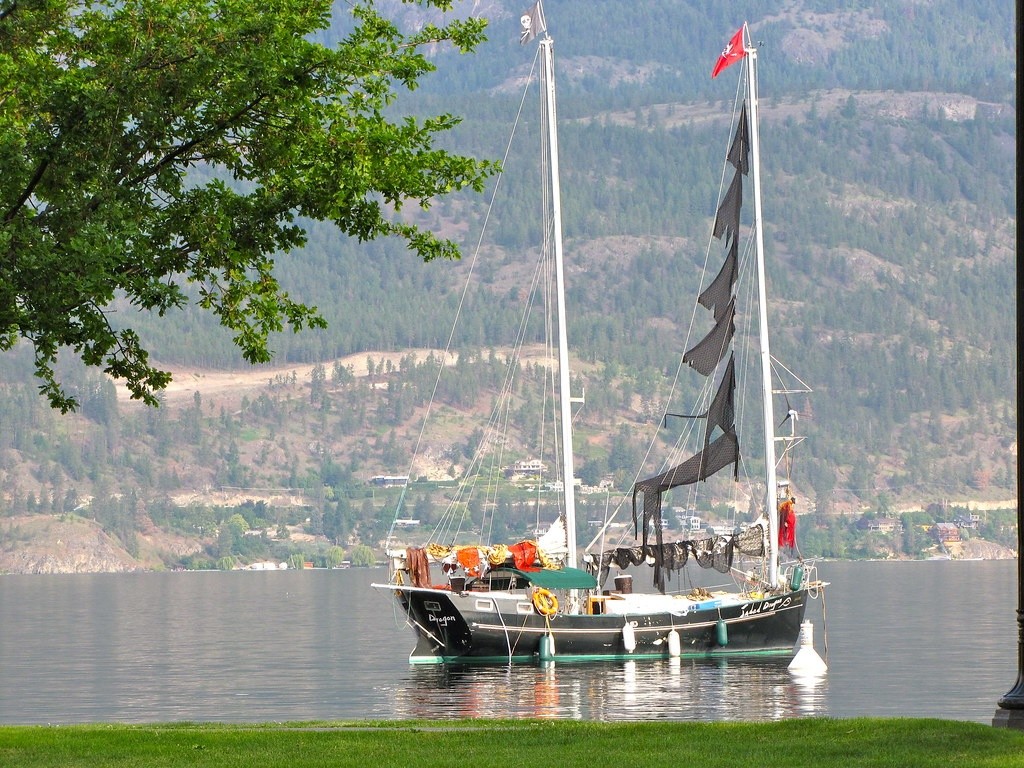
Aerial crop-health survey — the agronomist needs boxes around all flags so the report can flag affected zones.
[712,23,749,78]
[519,0,547,46]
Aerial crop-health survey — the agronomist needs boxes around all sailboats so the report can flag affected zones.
[369,0,832,667]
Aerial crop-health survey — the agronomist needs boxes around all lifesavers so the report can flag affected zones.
[533,588,558,615]
